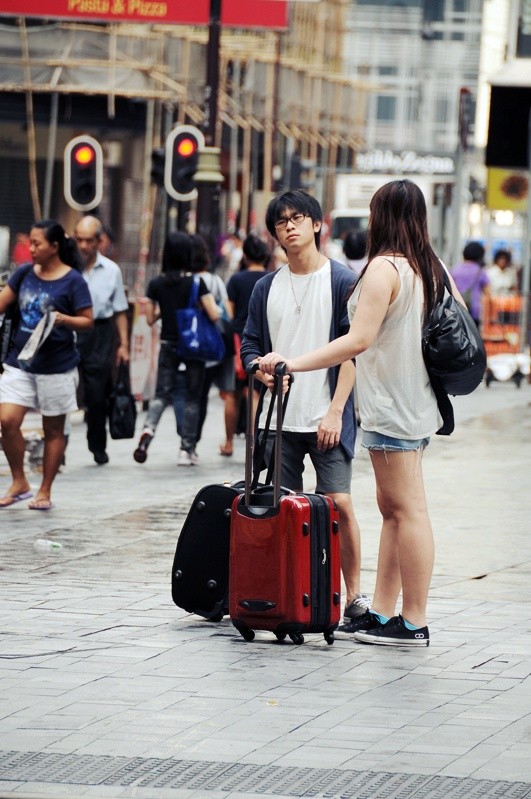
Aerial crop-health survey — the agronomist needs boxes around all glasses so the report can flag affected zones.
[274,212,306,229]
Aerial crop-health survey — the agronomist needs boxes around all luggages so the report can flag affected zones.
[169,371,293,621]
[227,361,342,647]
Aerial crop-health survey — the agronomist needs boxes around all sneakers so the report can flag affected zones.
[333,608,385,642]
[343,593,372,624]
[353,616,430,647]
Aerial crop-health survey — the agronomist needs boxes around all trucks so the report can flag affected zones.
[325,175,456,268]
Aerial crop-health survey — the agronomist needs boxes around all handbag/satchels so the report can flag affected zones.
[420,256,488,394]
[174,275,225,362]
[0,263,32,363]
[109,358,137,440]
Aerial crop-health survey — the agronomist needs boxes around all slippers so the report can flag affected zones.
[0,491,33,507]
[28,498,56,509]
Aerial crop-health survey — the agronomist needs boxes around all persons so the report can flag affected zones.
[256,180,470,647]
[0,219,94,510]
[57,216,528,476]
[238,188,373,625]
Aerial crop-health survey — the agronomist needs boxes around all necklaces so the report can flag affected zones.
[288,251,321,315]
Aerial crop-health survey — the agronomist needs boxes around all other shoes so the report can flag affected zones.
[132,427,154,463]
[94,450,108,464]
[177,449,202,465]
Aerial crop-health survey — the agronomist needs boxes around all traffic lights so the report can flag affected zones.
[150,148,165,185]
[164,125,205,201]
[290,153,315,189]
[63,135,103,212]
